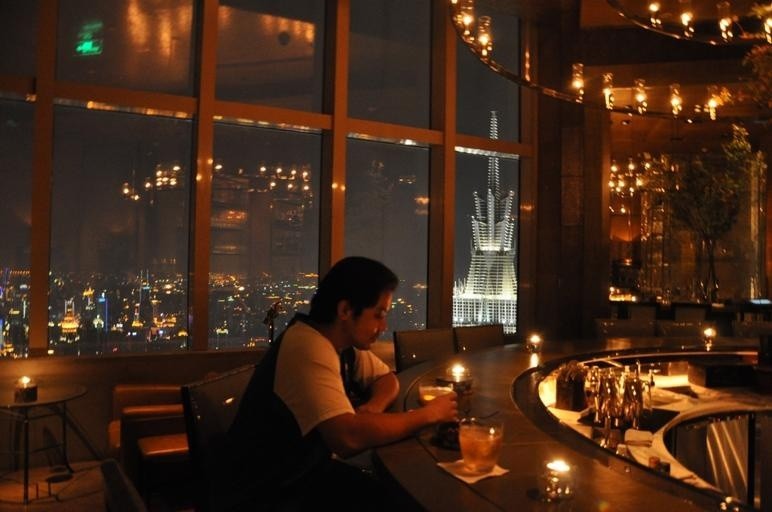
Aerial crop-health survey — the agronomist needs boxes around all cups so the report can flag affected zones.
[537,457,581,502]
[420,381,454,407]
[445,364,472,393]
[458,416,505,475]
[525,337,544,355]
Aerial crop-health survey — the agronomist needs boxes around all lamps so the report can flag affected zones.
[450,0,772,125]
[15,375,37,402]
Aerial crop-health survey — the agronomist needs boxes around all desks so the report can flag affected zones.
[0,373,103,505]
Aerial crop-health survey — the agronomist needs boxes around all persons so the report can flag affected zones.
[213,254,462,511]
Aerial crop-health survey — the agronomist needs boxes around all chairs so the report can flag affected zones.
[392,323,506,374]
[733,321,772,339]
[594,307,718,340]
[99,363,256,512]
